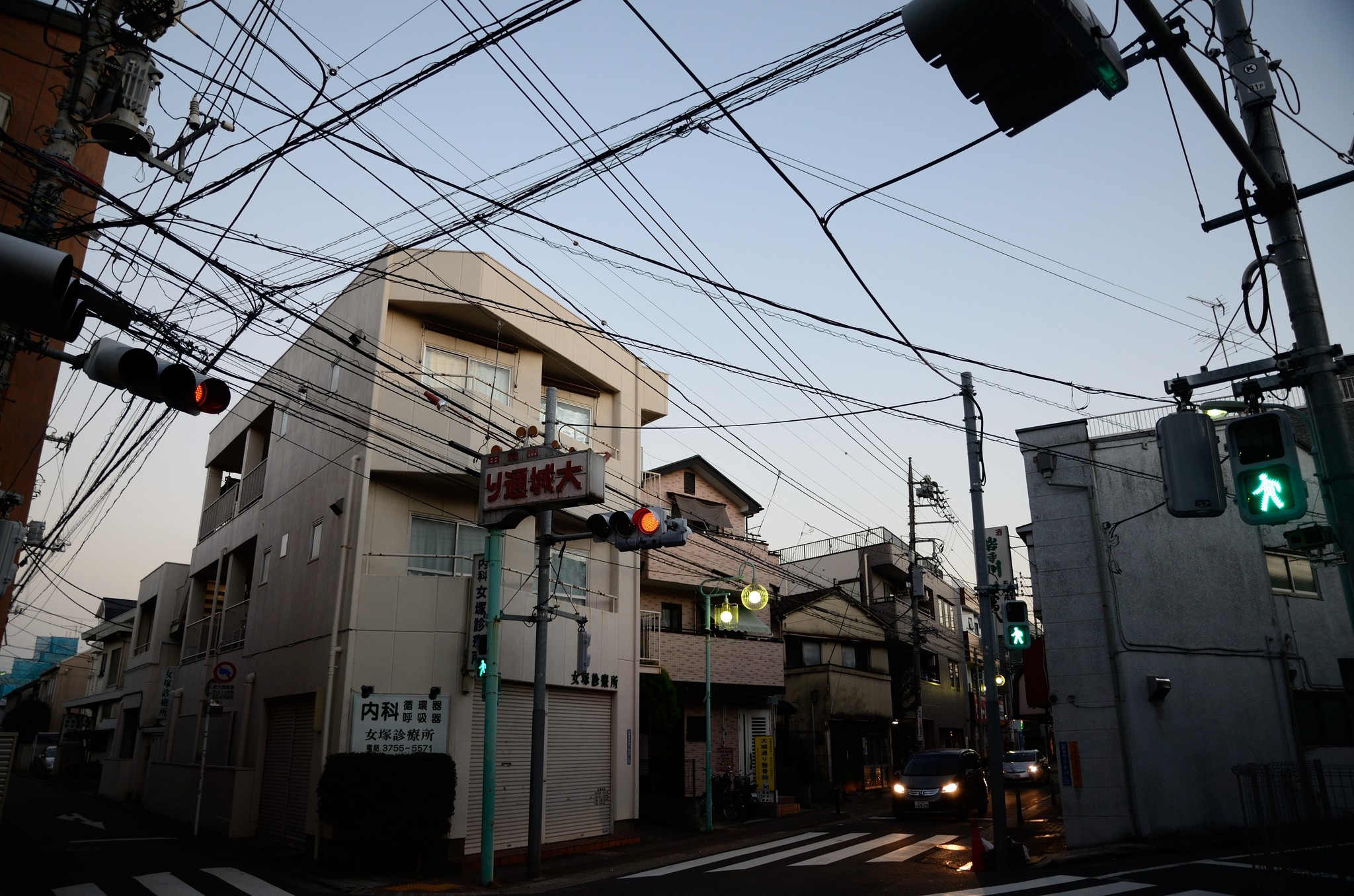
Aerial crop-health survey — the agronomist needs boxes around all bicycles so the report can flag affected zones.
[700,765,761,823]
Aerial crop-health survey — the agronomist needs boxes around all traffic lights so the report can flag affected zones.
[1002,600,1031,649]
[901,1,1127,137]
[585,507,667,540]
[577,631,591,674]
[82,336,232,418]
[474,633,487,676]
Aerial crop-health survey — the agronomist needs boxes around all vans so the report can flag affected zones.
[892,748,989,823]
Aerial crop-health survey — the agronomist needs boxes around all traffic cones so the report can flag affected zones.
[968,818,988,871]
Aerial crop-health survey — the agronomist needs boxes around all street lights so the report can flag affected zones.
[698,561,770,832]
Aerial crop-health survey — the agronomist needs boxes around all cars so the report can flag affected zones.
[34,746,56,776]
[1000,749,1051,784]
